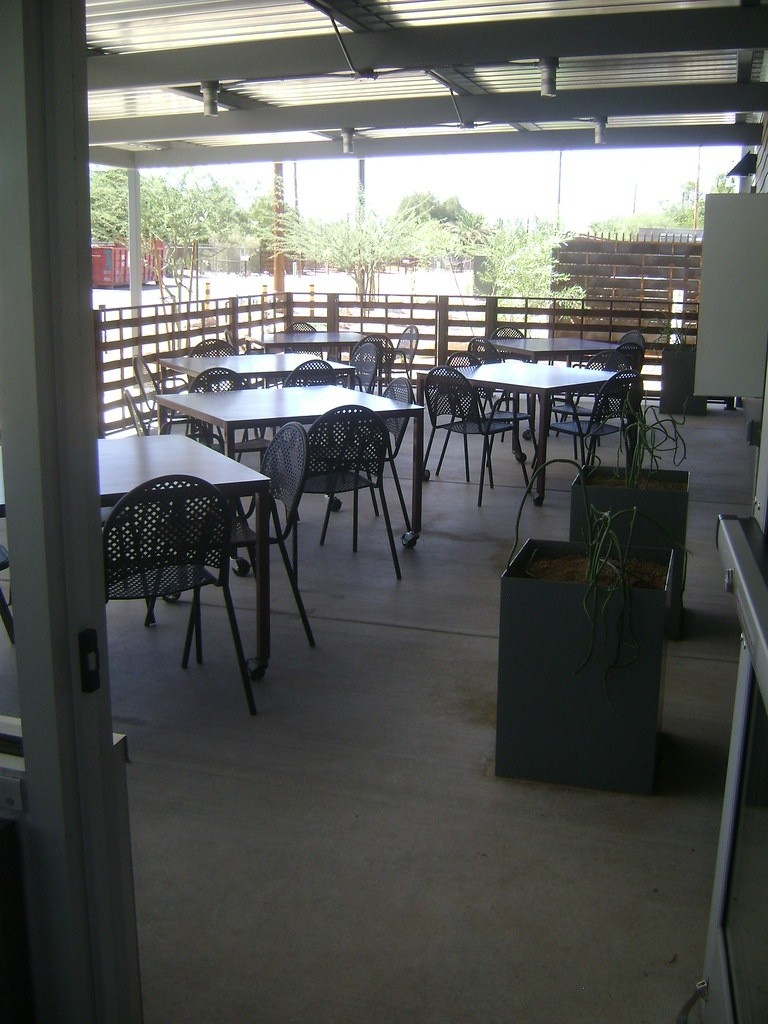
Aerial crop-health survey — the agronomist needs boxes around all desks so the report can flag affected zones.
[154,352,358,433]
[414,364,639,505]
[155,384,426,550]
[475,339,637,462]
[92,434,272,683]
[245,331,383,392]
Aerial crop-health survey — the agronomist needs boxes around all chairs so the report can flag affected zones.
[0,325,648,715]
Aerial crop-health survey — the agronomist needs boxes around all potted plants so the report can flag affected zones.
[497,453,677,797]
[645,300,707,417]
[568,391,692,641]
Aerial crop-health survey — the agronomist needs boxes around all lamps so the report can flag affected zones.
[727,151,758,177]
[200,80,220,117]
[594,115,608,145]
[459,121,478,130]
[341,126,356,154]
[538,57,559,97]
[350,68,378,82]
